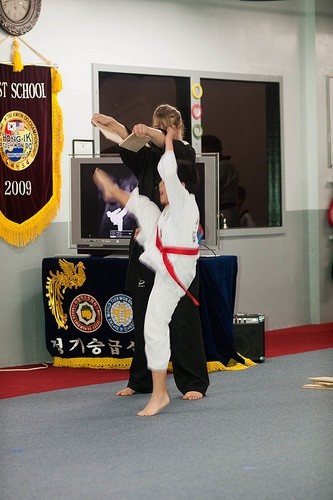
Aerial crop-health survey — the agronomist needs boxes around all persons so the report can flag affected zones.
[91,102,208,403]
[94,127,199,419]
[196,131,248,231]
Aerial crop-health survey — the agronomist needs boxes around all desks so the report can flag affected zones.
[41,254,254,376]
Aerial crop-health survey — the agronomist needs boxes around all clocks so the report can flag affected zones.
[0,0,41,36]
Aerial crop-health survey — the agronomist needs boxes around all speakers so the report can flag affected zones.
[232,312,265,362]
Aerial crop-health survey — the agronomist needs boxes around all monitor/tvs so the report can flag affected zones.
[67,154,221,252]
